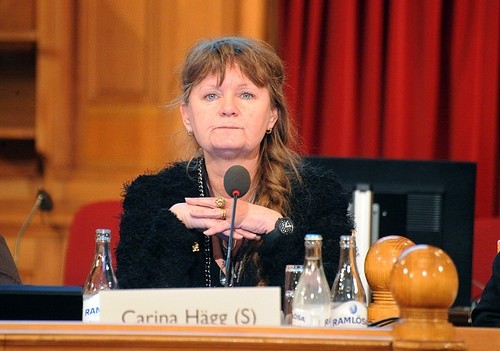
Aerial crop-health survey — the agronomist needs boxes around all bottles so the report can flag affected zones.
[330,235,368,329]
[83,229,118,294]
[292,233,331,328]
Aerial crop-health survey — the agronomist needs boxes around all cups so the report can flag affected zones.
[284,265,304,327]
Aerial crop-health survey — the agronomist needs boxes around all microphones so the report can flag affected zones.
[223,165,250,286]
[12,189,53,264]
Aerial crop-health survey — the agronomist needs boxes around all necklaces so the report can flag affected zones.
[222,187,257,250]
[198,156,212,288]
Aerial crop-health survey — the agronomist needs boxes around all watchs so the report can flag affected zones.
[261,216,296,244]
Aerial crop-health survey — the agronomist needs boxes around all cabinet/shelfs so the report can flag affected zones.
[1,0,77,185]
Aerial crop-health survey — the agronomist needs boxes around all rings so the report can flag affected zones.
[216,196,225,209]
[221,210,226,220]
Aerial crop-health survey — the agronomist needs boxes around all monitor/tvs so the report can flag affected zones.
[294,155,473,313]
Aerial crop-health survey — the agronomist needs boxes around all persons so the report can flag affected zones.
[471,250,500,329]
[114,36,355,316]
